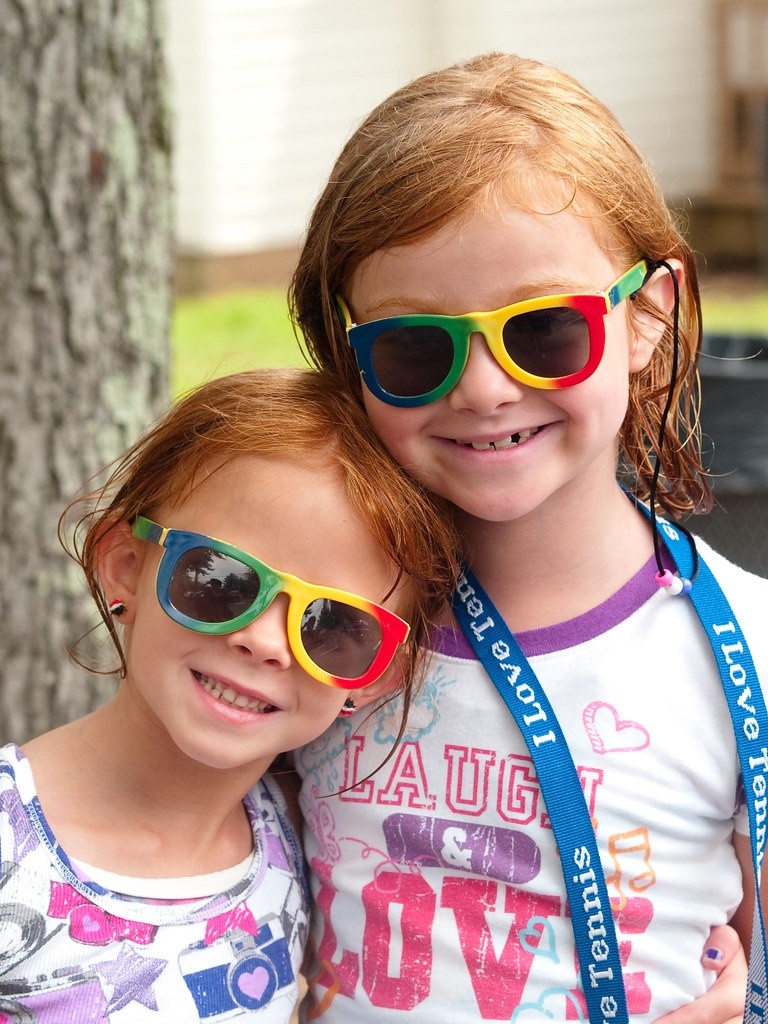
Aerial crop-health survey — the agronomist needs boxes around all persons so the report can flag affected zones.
[1,369,752,1024]
[293,55,767,1024]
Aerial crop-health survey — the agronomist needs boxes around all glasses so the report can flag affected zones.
[130,514,410,691]
[337,259,647,409]
